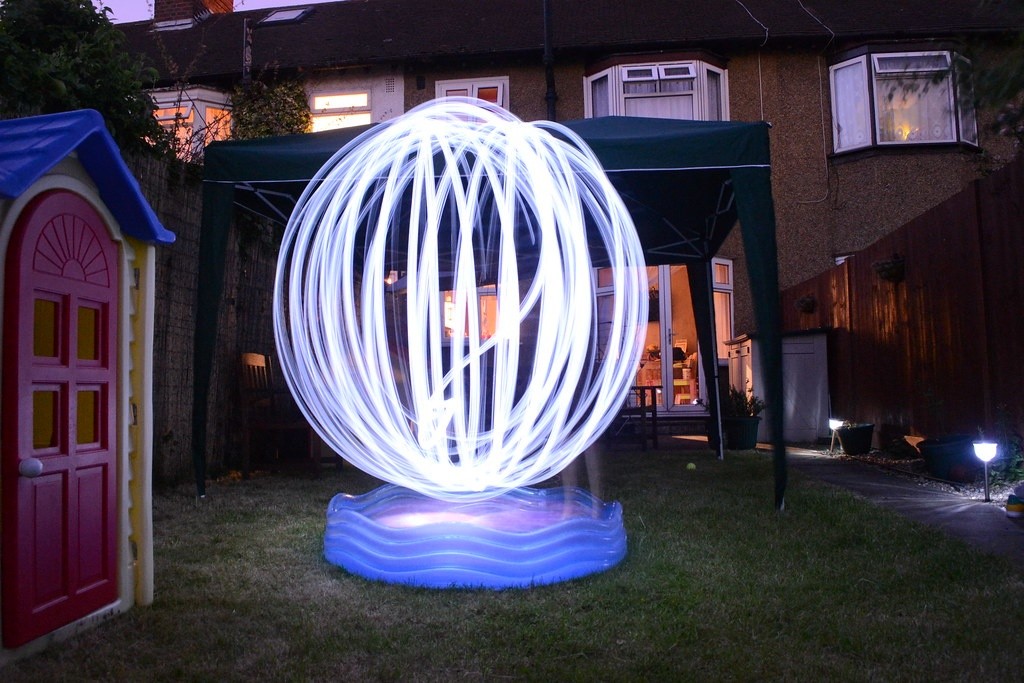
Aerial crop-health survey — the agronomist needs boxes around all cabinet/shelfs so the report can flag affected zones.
[722,327,839,443]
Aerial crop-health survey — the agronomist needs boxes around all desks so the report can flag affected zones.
[604,386,663,451]
[637,361,682,407]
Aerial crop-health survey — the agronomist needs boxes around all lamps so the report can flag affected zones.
[272,96,649,591]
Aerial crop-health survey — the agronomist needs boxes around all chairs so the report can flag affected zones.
[673,352,698,405]
[235,352,321,479]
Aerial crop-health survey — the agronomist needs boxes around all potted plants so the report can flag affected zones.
[695,384,768,450]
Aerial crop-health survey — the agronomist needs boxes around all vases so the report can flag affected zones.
[917,435,980,484]
[836,423,875,456]
